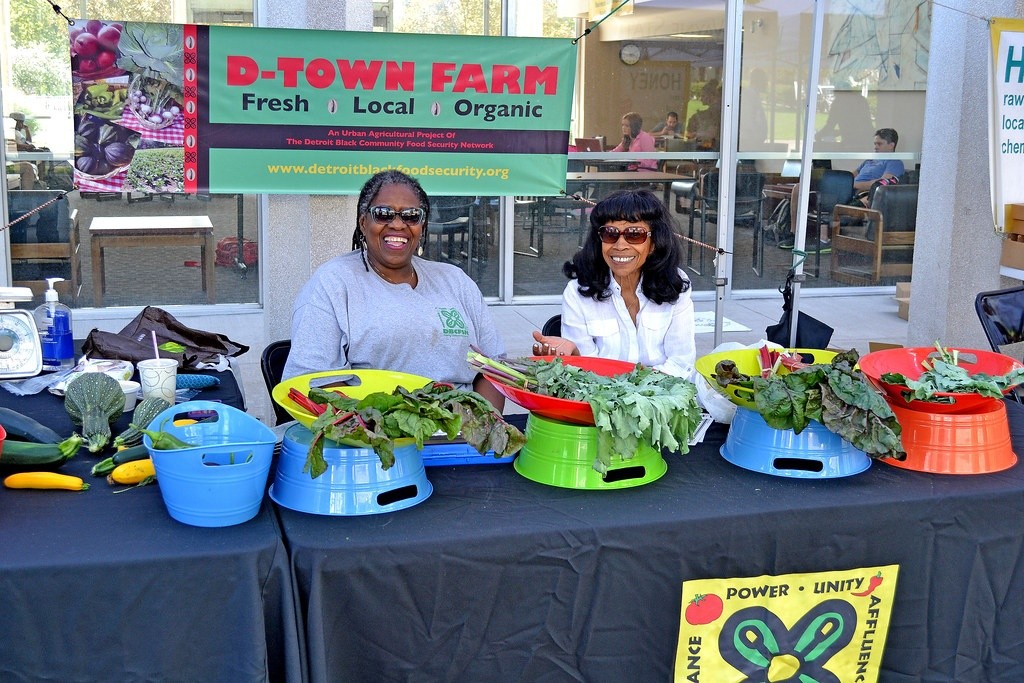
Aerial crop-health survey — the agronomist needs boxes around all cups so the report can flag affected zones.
[137,358,179,408]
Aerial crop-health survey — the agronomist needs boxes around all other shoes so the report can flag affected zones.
[804,236,832,254]
[777,233,795,248]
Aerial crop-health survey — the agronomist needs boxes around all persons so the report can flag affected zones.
[533,189,696,382]
[611,112,657,171]
[649,112,684,135]
[813,81,872,145]
[779,127,904,253]
[280,169,506,419]
[741,69,768,144]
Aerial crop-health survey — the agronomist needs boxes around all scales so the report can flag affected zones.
[0,287,44,381]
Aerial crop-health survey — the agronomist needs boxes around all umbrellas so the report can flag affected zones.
[217,237,258,279]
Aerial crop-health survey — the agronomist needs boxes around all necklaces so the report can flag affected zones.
[369,260,413,285]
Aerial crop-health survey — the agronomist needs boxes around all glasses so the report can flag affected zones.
[365,205,425,226]
[598,225,652,244]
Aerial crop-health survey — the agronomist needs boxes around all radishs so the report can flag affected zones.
[132,91,180,125]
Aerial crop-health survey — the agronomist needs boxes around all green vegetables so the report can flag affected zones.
[286,341,1024,478]
[115,21,186,91]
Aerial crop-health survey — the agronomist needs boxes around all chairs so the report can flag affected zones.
[829,184,919,286]
[431,138,854,281]
[976,285,1024,408]
[7,189,82,304]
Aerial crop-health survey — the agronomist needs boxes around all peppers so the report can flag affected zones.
[129,422,195,451]
[82,84,129,119]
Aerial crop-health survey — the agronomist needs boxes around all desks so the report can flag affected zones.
[272,449,1024,683]
[0,339,300,683]
[89,216,214,297]
[528,171,696,255]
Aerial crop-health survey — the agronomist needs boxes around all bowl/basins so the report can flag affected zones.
[118,380,141,412]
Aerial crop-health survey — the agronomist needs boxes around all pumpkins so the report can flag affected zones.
[64,372,171,451]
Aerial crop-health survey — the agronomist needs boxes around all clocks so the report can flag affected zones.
[619,43,641,66]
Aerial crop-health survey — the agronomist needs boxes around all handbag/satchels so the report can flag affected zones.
[216,235,258,267]
[82,304,251,412]
